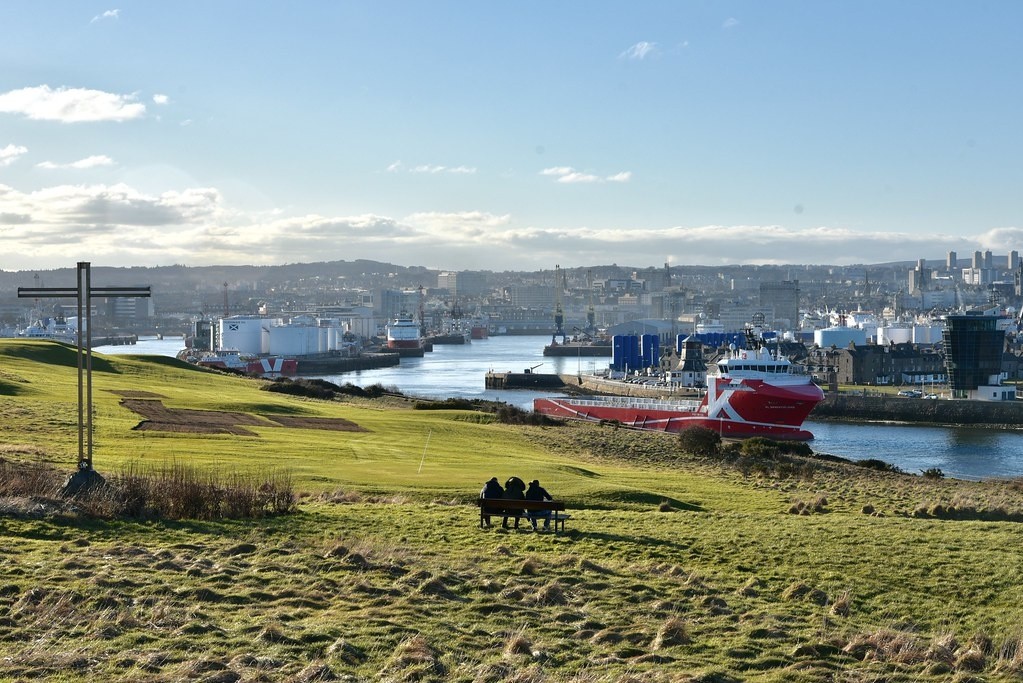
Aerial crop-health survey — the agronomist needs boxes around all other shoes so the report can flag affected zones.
[503,525,519,530]
[532,526,550,531]
[487,524,494,527]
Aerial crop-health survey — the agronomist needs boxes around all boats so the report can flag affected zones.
[534,342,826,445]
[19,316,78,346]
[384,305,422,349]
[198,348,298,380]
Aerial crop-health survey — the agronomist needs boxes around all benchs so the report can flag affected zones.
[476,497,570,534]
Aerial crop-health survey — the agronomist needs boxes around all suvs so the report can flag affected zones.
[897,390,926,398]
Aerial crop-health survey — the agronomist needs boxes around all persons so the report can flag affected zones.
[526,480,555,531]
[480,477,509,528]
[504,477,530,528]
